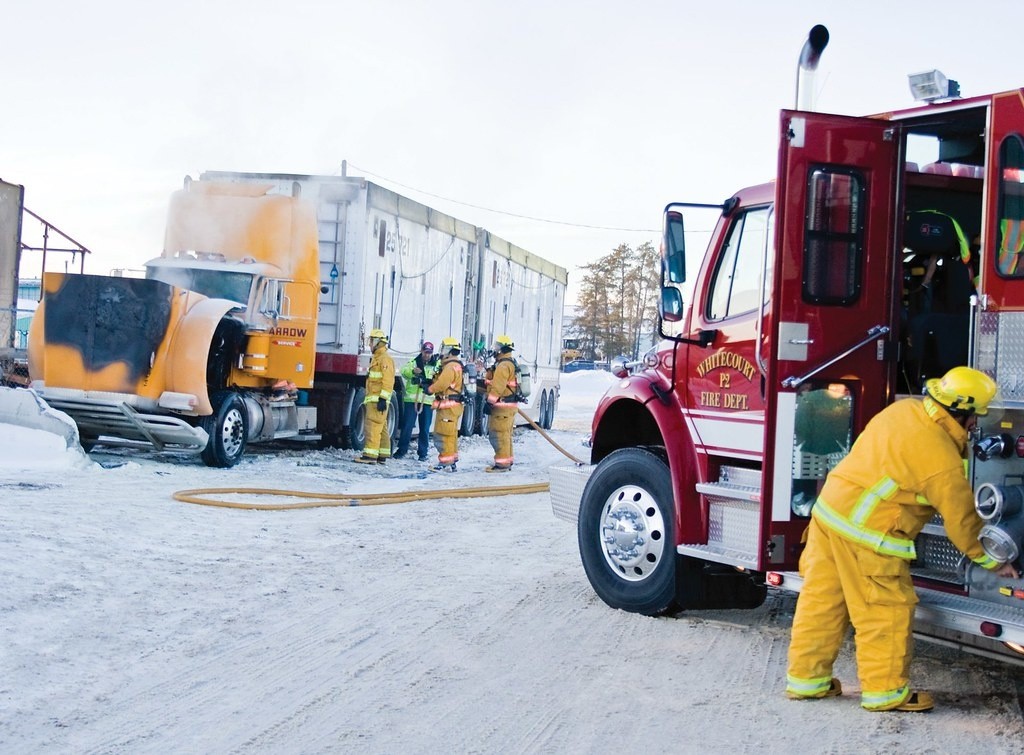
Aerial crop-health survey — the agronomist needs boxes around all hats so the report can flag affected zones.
[422,343,433,353]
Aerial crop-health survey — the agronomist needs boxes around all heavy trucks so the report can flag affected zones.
[24,169,570,469]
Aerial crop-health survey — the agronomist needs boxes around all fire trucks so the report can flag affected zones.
[578,22,1023,717]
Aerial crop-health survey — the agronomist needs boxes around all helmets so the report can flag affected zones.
[925,366,997,415]
[442,339,462,351]
[369,329,388,345]
[497,336,515,349]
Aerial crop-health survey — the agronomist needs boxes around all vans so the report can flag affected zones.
[560,360,594,373]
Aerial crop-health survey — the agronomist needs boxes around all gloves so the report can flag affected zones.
[377,399,387,412]
[422,387,433,396]
[483,402,492,415]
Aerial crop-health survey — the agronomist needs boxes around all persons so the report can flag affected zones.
[475,335,517,472]
[422,339,463,472]
[393,342,442,461]
[787,367,1019,710]
[355,330,395,464]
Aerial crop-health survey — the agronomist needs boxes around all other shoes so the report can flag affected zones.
[895,691,934,712]
[378,457,386,463]
[823,678,842,698]
[393,451,403,459]
[354,453,377,464]
[420,455,427,461]
[429,462,456,473]
[486,463,510,472]
